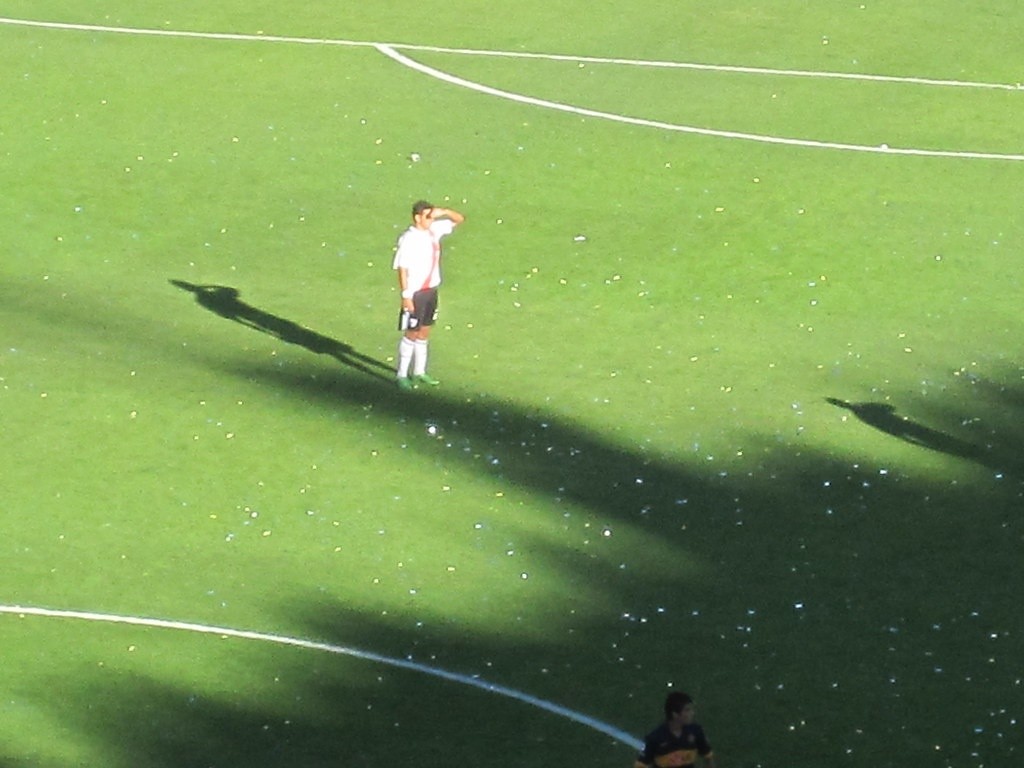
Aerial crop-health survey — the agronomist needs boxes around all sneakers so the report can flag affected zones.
[396,376,418,392]
[413,373,440,385]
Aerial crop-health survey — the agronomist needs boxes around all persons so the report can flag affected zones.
[633,692,717,768]
[384,201,465,391]
